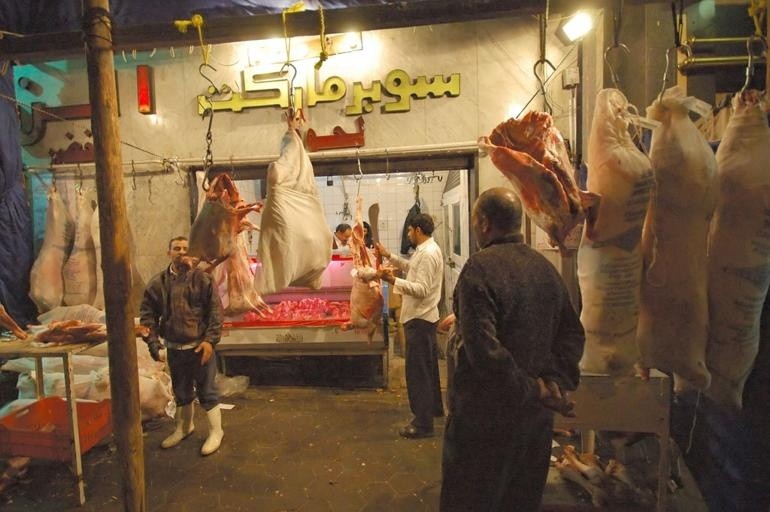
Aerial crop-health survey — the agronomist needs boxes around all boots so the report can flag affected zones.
[200,402,225,456]
[160,400,195,449]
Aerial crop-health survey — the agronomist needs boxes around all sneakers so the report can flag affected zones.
[399,423,436,438]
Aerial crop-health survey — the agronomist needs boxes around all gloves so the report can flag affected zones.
[148,341,164,363]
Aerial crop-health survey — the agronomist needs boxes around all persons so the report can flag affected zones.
[363,220,374,248]
[331,222,353,255]
[369,212,446,438]
[438,186,586,512]
[140,236,226,456]
